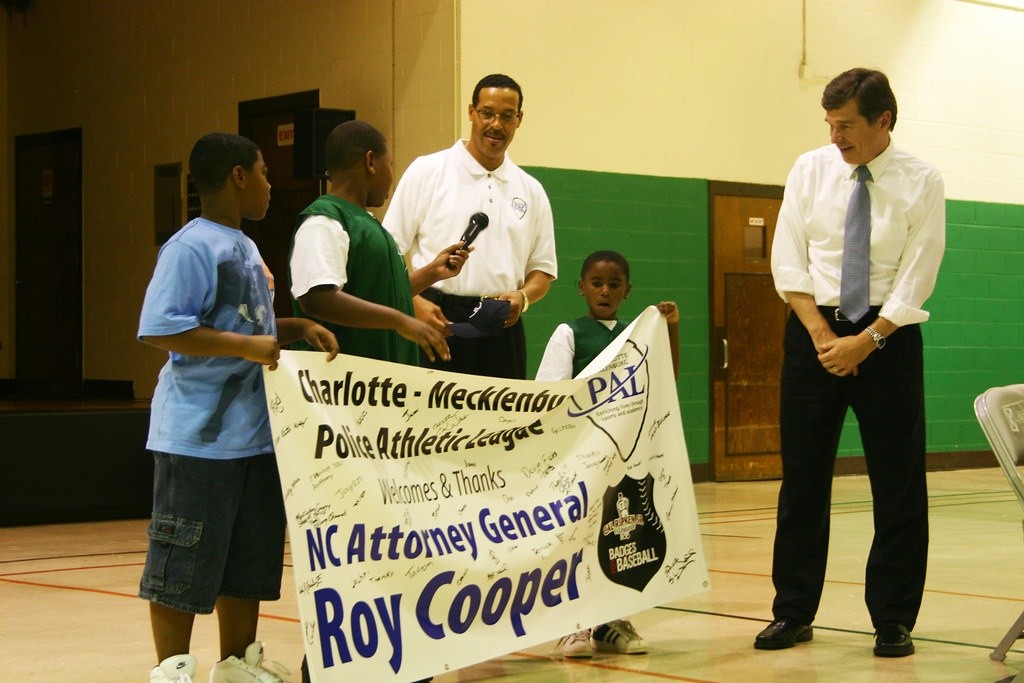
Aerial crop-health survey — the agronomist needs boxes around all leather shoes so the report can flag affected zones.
[753,620,814,649]
[873,626,914,658]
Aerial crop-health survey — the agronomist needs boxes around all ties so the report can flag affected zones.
[839,165,871,323]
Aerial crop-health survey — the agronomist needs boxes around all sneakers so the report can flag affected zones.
[555,629,593,658]
[209,641,291,683]
[150,654,198,683]
[589,618,648,653]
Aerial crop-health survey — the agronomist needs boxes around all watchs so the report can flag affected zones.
[865,326,886,349]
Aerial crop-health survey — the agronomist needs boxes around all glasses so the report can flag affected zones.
[472,104,520,123]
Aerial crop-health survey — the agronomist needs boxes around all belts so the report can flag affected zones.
[816,305,881,323]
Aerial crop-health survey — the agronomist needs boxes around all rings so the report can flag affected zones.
[833,367,837,372]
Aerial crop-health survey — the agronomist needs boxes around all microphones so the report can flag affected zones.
[445,212,489,270]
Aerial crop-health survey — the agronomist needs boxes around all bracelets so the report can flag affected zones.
[517,290,529,314]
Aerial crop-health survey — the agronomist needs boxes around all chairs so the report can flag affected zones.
[972,383,1024,661]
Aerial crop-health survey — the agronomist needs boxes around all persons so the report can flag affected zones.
[287,120,475,683]
[536,250,680,657]
[754,69,947,657]
[138,134,340,683]
[381,74,558,380]
